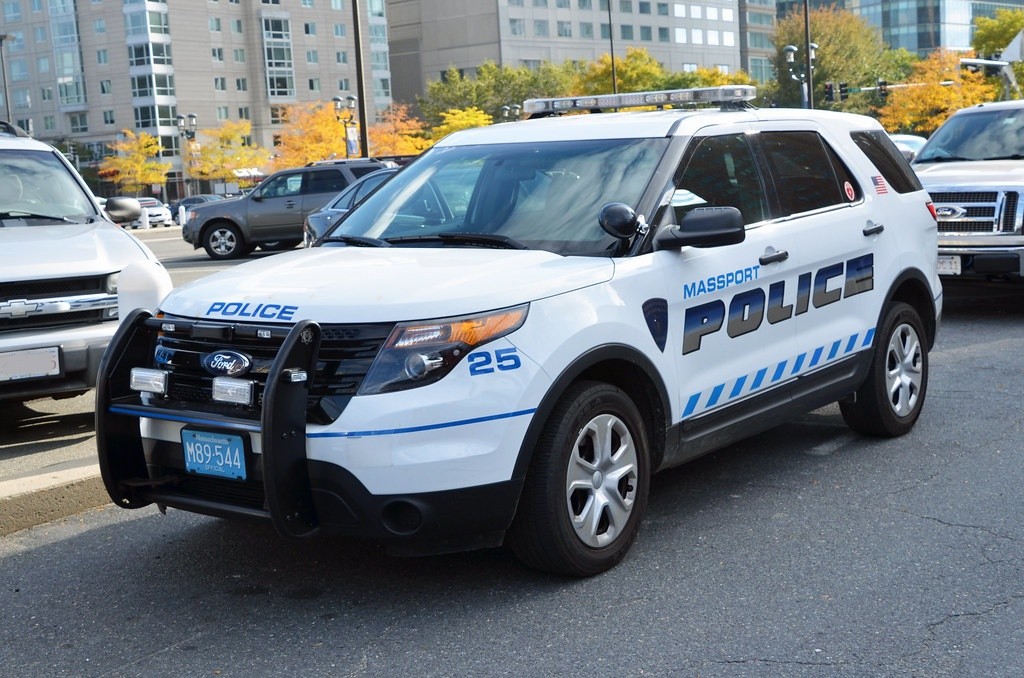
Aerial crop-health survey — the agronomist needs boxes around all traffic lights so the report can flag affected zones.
[877,80,889,98]
[824,83,834,102]
[839,82,850,101]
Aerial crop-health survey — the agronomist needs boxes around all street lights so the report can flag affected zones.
[784,43,820,110]
[332,95,357,160]
[0,33,16,124]
[176,113,198,195]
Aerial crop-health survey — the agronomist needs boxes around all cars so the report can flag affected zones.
[812,133,954,180]
[130,197,172,229]
[301,164,565,250]
[167,194,224,225]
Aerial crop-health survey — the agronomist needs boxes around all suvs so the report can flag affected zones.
[182,157,408,261]
[907,101,1024,299]
[93,86,945,582]
[0,120,174,400]
